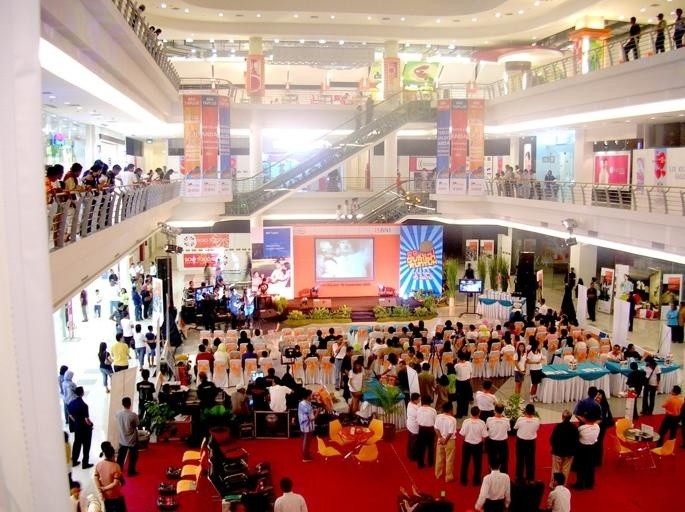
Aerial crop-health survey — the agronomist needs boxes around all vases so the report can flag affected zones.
[625,398,635,428]
[136,430,151,448]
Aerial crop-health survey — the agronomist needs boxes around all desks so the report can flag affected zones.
[185,385,226,415]
[478,294,527,324]
[626,428,660,451]
[342,425,373,460]
[608,361,684,397]
[361,375,408,432]
[539,363,610,402]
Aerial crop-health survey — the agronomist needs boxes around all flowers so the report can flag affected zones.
[619,389,637,398]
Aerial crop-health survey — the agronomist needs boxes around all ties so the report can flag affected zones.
[76,501,82,512]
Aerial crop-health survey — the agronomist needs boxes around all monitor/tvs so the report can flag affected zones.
[459,279,484,294]
[196,286,214,301]
[250,372,263,381]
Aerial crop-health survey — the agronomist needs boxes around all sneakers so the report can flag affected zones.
[108,315,115,321]
[416,463,426,469]
[81,463,93,470]
[139,367,144,371]
[472,479,481,485]
[529,395,534,403]
[454,413,462,419]
[445,476,455,483]
[428,462,433,468]
[128,355,132,359]
[127,470,140,477]
[135,355,138,358]
[533,395,538,402]
[460,477,468,487]
[72,461,80,467]
[435,472,443,480]
[105,390,110,393]
[148,364,157,368]
[302,456,313,463]
[81,319,89,322]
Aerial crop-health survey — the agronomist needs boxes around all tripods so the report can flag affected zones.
[427,342,443,378]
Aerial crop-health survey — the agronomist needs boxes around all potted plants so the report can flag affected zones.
[444,259,459,317]
[144,400,174,440]
[497,392,540,435]
[204,405,232,444]
[366,379,406,442]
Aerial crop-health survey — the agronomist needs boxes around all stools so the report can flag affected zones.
[253,410,289,440]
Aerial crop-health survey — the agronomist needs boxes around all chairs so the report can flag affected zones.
[209,454,269,480]
[207,435,249,461]
[176,465,201,496]
[348,324,517,378]
[609,430,632,461]
[329,420,351,446]
[649,438,676,468]
[616,418,637,443]
[314,436,341,460]
[206,466,272,502]
[517,315,613,362]
[183,437,206,463]
[181,451,205,480]
[354,445,379,464]
[366,419,383,444]
[173,320,346,383]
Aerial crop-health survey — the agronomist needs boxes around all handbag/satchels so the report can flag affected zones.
[104,352,112,365]
[644,366,656,387]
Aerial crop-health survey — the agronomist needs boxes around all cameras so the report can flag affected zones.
[278,340,300,359]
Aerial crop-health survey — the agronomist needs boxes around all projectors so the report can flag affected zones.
[378,284,387,296]
[310,286,318,293]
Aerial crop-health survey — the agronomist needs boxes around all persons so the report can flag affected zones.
[44,157,58,255]
[417,396,437,468]
[586,334,600,347]
[182,277,269,328]
[55,363,69,395]
[320,337,330,350]
[421,168,429,193]
[550,410,579,490]
[196,329,272,367]
[566,268,576,298]
[442,278,450,294]
[80,160,174,233]
[353,105,363,129]
[120,312,138,359]
[110,333,132,372]
[146,28,161,55]
[573,387,603,424]
[349,360,364,413]
[94,289,102,318]
[655,385,684,449]
[110,303,124,332]
[246,377,270,408]
[493,164,557,199]
[68,387,97,470]
[644,356,661,416]
[400,484,435,512]
[252,261,295,299]
[306,344,320,360]
[325,329,336,341]
[262,368,275,385]
[459,407,489,486]
[136,369,155,430]
[625,343,642,361]
[352,197,361,224]
[232,384,252,422]
[314,329,323,341]
[513,404,540,484]
[94,447,128,512]
[672,8,685,50]
[434,403,458,482]
[535,299,587,360]
[200,255,229,277]
[598,159,612,188]
[298,390,322,463]
[157,384,179,405]
[266,377,295,413]
[418,363,436,405]
[585,283,598,321]
[343,198,352,224]
[109,269,118,284]
[513,336,522,345]
[443,363,458,417]
[469,320,512,376]
[70,481,90,512]
[314,241,368,280]
[595,390,612,467]
[474,457,510,512]
[124,305,130,320]
[366,95,375,125]
[513,342,528,404]
[460,281,477,291]
[335,204,344,225]
[605,343,625,363]
[293,345,304,359]
[666,302,679,343]
[57,181,73,248]
[274,478,307,512]
[454,353,472,418]
[128,261,157,320]
[97,441,112,458]
[115,397,143,477]
[197,372,219,409]
[57,370,78,425]
[436,375,451,414]
[145,325,156,369]
[396,174,406,196]
[512,311,523,322]
[575,279,584,298]
[53,164,64,246]
[527,340,543,403]
[678,302,685,343]
[340,346,354,402]
[65,432,74,480]
[128,4,146,32]
[118,289,129,304]
[622,18,642,62]
[525,334,534,351]
[332,335,349,391]
[405,392,421,460]
[524,316,534,329]
[365,319,470,370]
[655,14,666,54]
[149,26,154,32]
[546,472,570,512]
[65,164,92,242]
[486,405,512,475]
[79,290,88,322]
[463,263,474,297]
[133,324,147,370]
[357,356,369,380]
[626,291,638,331]
[578,411,600,491]
[396,361,411,392]
[98,343,115,394]
[626,363,647,423]
[109,280,119,313]
[476,381,498,420]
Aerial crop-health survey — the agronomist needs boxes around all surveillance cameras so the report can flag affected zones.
[165,244,183,254]
[559,238,577,248]
